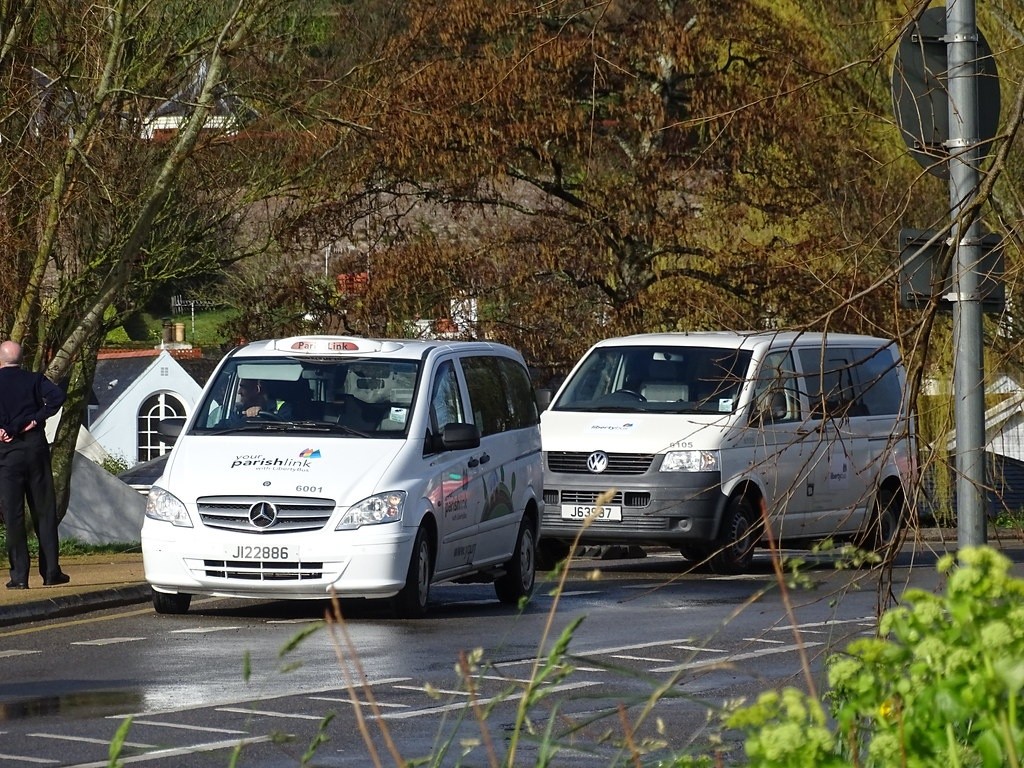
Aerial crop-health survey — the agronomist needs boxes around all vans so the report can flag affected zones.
[537,331,917,578]
[140,331,548,617]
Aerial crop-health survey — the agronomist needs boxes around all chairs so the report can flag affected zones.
[636,358,688,406]
[730,357,786,413]
[228,375,460,438]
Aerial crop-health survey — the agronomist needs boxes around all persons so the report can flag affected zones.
[0,341,71,589]
[215,378,295,429]
